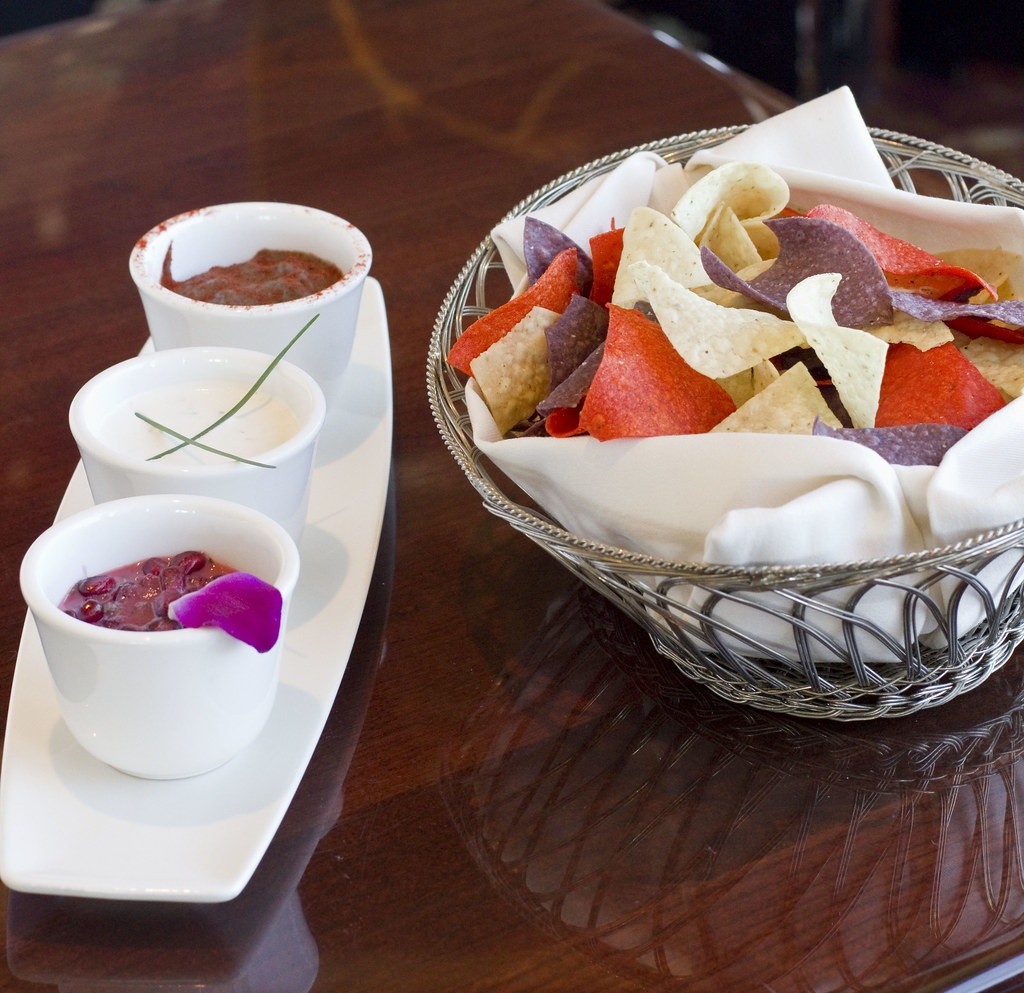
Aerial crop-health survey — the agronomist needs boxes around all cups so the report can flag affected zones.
[69,349,324,554]
[129,202,372,410]
[19,493,300,781]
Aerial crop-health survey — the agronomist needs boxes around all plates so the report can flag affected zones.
[1,275,392,906]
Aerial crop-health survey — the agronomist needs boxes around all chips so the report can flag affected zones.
[445,161,1024,467]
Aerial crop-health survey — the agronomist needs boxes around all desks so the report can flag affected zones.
[0,0,1024,993]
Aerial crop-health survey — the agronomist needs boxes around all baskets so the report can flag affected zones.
[427,119,1024,719]
